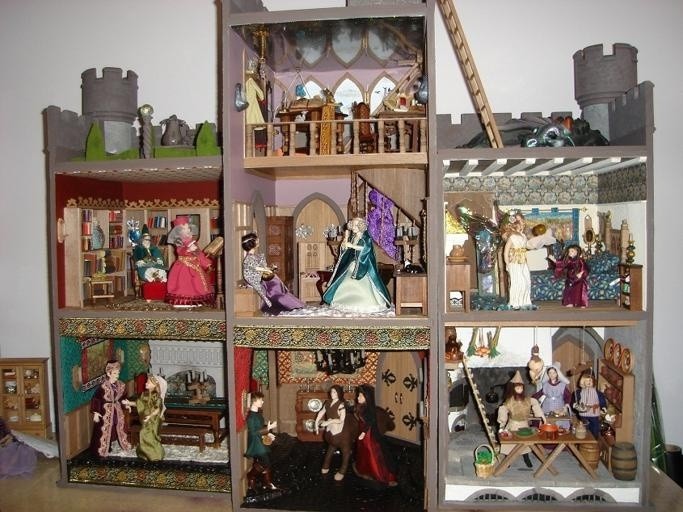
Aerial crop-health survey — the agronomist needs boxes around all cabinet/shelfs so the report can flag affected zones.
[0,358,53,440]
[43,0,654,512]
